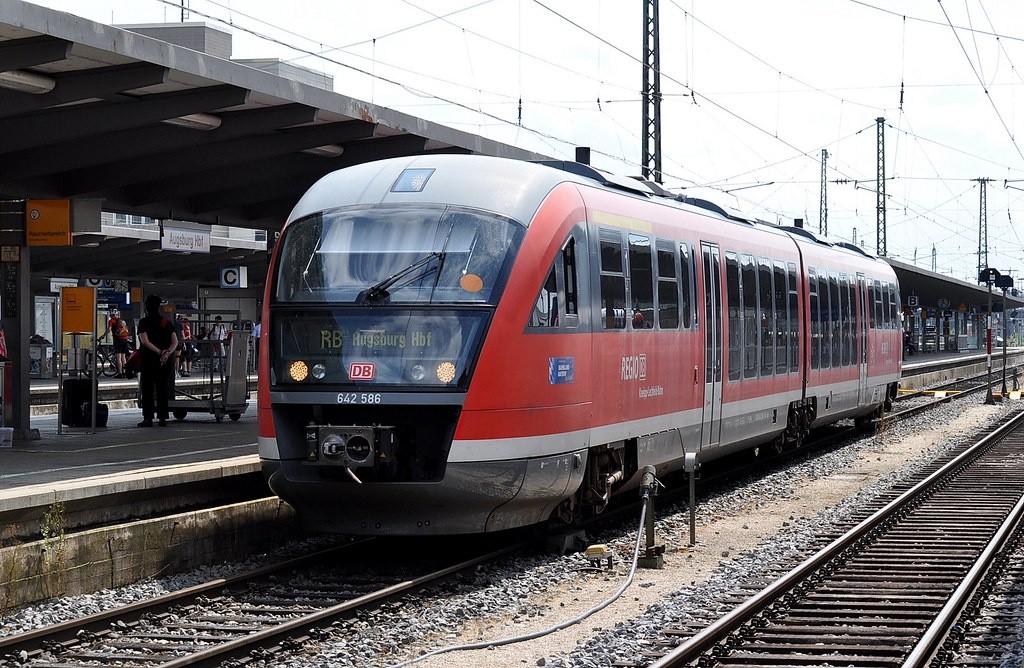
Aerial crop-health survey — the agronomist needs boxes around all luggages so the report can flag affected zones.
[79,402,108,427]
[60,379,99,426]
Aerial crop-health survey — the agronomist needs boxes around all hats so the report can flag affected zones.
[110,308,119,315]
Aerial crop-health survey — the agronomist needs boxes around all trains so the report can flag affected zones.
[255,155,902,534]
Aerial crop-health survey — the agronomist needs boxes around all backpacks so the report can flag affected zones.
[112,318,128,337]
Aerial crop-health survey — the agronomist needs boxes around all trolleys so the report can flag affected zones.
[167,319,257,422]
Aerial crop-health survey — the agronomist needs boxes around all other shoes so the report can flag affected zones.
[115,373,127,379]
[182,372,191,377]
[175,372,182,378]
[903,358,908,361]
[137,419,154,427]
[159,420,167,427]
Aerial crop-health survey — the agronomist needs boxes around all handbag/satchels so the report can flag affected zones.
[124,349,144,379]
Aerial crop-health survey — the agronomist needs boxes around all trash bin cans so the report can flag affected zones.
[63,379,99,427]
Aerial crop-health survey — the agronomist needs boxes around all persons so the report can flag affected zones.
[181,317,192,376]
[137,296,179,426]
[175,315,186,378]
[902,321,907,361]
[906,331,917,353]
[634,303,645,329]
[208,316,227,372]
[250,323,261,353]
[195,326,208,353]
[99,308,128,379]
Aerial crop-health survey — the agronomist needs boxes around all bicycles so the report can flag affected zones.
[82,339,122,377]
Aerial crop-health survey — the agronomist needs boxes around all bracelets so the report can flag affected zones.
[160,350,162,356]
